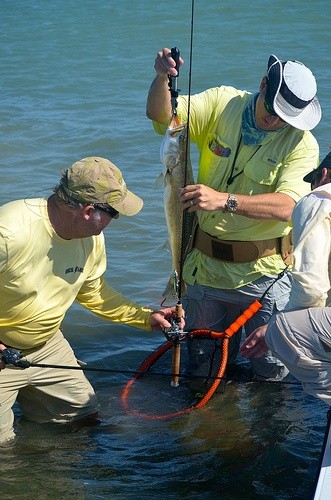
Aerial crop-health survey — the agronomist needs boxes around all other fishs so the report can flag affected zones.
[159,114,196,299]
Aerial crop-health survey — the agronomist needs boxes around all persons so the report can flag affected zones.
[0,156,186,446]
[147,49,321,382]
[239,151,331,407]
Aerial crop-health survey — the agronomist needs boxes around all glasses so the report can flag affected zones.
[96,207,119,220]
[264,98,286,123]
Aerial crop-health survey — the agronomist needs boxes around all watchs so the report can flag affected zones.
[224,192,238,214]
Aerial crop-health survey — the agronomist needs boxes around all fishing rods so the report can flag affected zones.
[161,0,195,387]
[0,348,302,386]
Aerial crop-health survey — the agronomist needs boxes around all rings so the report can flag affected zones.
[190,200,195,206]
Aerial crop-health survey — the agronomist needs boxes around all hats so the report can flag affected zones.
[268,54,322,132]
[60,156,143,217]
[303,152,331,182]
